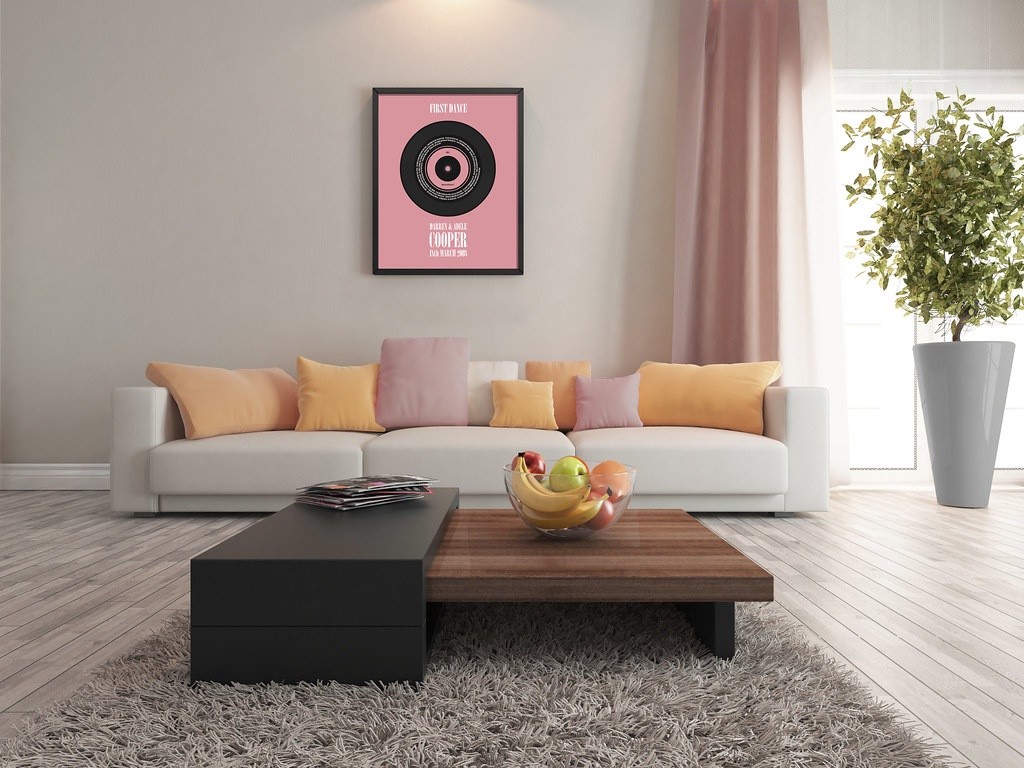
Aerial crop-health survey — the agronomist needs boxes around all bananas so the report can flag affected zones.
[513,452,611,528]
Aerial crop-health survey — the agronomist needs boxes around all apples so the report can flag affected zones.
[549,457,588,493]
[512,451,545,482]
[587,493,614,529]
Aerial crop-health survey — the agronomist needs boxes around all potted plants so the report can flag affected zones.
[840,84,1024,507]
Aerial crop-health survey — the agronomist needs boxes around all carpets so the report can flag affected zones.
[1,601,973,767]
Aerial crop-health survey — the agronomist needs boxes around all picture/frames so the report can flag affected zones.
[372,86,525,275]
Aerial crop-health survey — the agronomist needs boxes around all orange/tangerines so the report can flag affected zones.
[592,461,629,502]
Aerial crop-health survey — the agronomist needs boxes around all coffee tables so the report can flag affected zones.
[191,487,776,690]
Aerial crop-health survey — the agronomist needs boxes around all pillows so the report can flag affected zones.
[489,380,559,429]
[525,360,591,429]
[377,336,469,428]
[573,371,643,431]
[146,361,300,439]
[469,360,519,425]
[638,360,783,435]
[295,356,386,432]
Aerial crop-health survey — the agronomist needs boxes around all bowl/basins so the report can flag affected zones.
[503,460,638,540]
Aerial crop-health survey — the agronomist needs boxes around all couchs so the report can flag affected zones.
[110,386,830,516]
